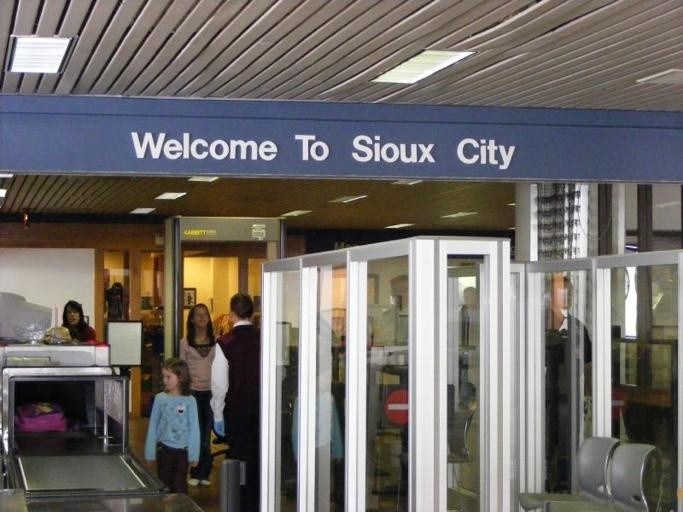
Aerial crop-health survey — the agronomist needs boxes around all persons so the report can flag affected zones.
[61,300,97,342]
[180,302,219,486]
[208,293,260,512]
[145,357,201,494]
[542,276,592,492]
[458,286,479,347]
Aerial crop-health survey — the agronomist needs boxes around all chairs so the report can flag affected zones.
[396,408,472,509]
[514,435,658,512]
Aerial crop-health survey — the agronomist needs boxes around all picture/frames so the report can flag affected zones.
[182,287,197,309]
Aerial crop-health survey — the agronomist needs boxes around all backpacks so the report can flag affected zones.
[14,401,69,433]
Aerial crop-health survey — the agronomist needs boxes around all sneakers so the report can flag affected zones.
[187,478,211,487]
